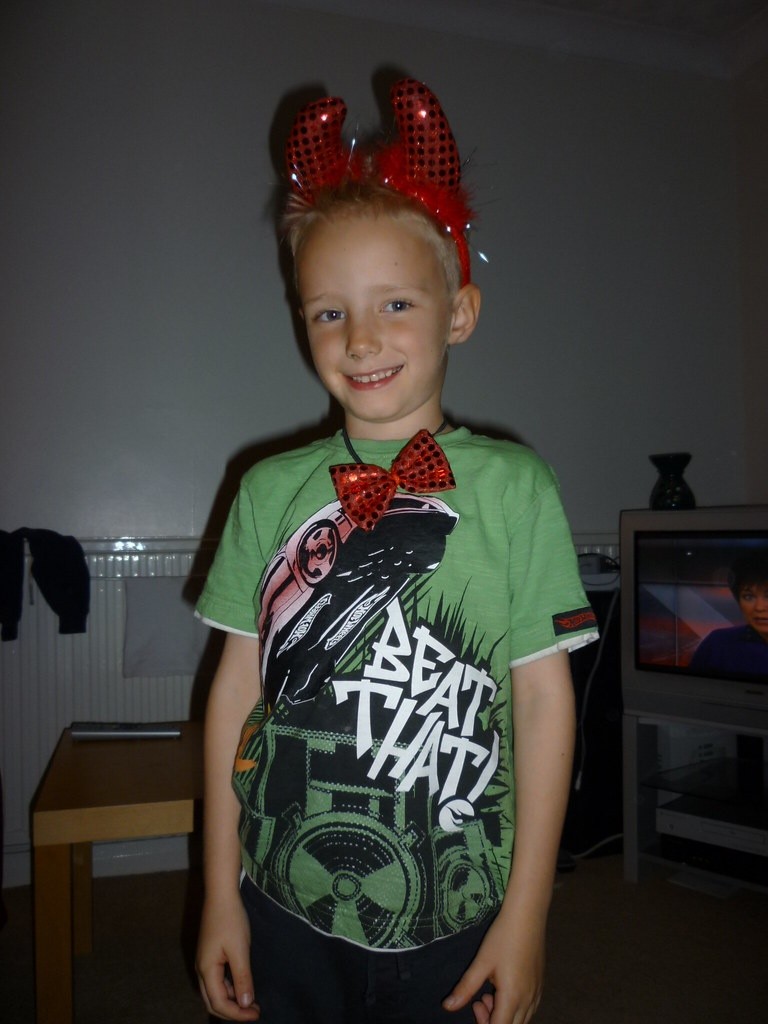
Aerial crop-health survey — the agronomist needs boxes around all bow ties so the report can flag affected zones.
[329,421,456,531]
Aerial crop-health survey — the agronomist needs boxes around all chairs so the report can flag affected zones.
[33,724,206,1024]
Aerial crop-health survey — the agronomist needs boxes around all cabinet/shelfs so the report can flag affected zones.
[623,694,768,885]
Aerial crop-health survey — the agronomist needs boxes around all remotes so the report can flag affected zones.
[70,720,181,742]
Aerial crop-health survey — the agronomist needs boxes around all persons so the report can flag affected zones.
[689,554,768,675]
[195,184,600,1024]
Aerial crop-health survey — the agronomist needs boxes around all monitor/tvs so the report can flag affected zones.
[616,505,768,730]
